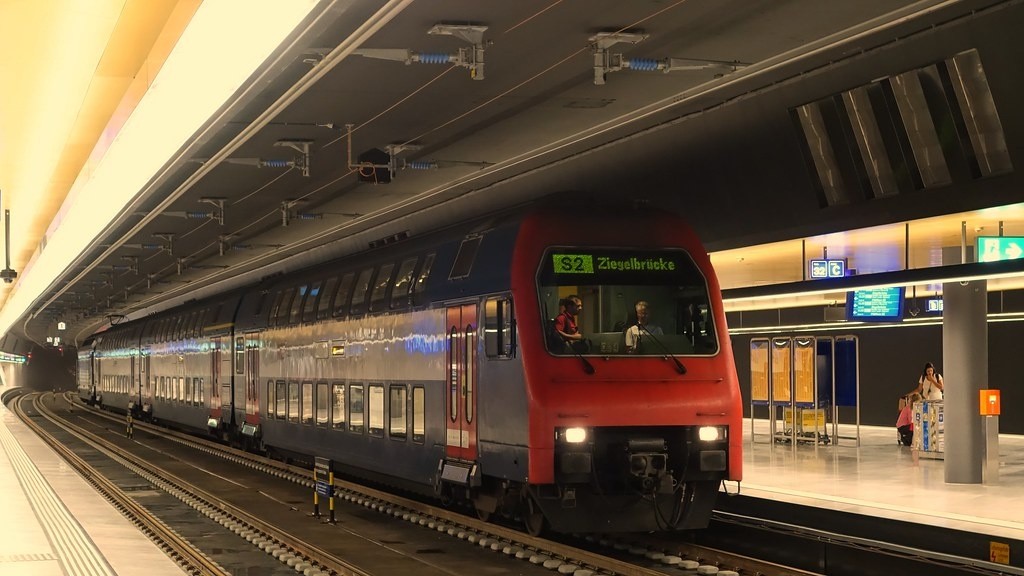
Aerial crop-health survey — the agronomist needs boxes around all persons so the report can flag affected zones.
[909,362,944,400]
[552,295,582,346]
[895,393,923,446]
[626,302,666,353]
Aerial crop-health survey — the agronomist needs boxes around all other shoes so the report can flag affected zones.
[901,437,910,446]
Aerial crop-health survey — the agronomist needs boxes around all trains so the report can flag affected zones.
[76,195,744,539]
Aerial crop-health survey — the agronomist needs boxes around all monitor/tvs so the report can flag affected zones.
[845,286,906,322]
